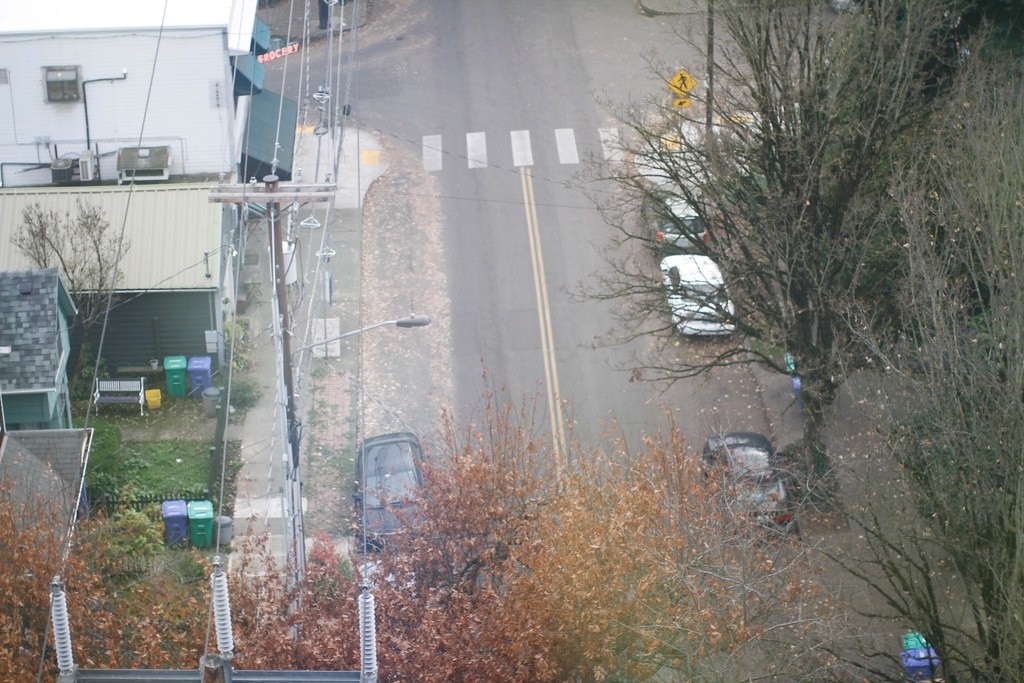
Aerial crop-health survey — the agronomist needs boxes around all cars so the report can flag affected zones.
[700,431,801,548]
[658,255,736,336]
[356,433,431,552]
[642,182,709,260]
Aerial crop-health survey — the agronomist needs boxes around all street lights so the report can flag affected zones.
[280,313,435,642]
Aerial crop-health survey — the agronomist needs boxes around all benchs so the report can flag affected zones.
[116,365,163,381]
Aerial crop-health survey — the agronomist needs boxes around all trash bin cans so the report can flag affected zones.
[900,631,940,683]
[783,352,805,409]
[162,500,187,548]
[188,356,211,397]
[201,387,220,418]
[145,389,162,411]
[187,500,213,548]
[163,355,187,398]
[214,516,233,545]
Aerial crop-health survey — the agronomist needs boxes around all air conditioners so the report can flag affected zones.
[79,150,96,180]
[121,152,169,180]
